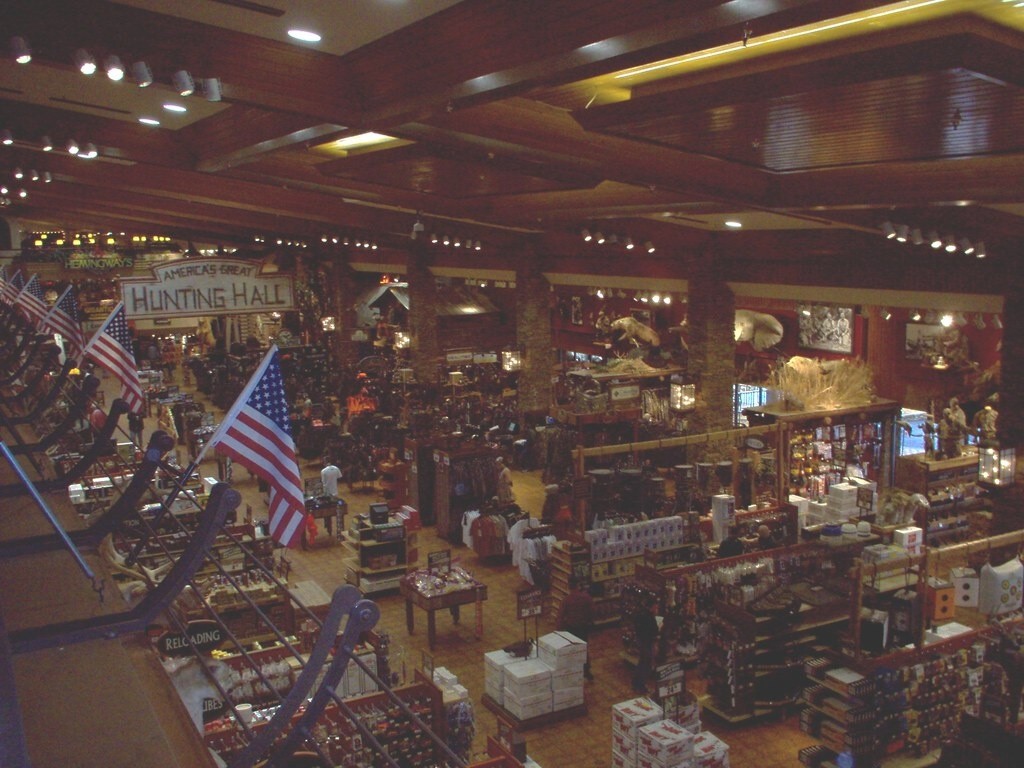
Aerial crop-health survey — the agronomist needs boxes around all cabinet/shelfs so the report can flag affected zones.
[340,531,358,572]
[754,605,850,707]
[549,557,577,615]
[590,553,648,625]
[361,538,411,573]
[802,674,866,753]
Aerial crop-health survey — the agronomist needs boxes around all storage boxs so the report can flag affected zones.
[235,473,923,768]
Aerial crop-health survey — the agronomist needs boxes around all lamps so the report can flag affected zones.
[580,229,656,254]
[881,222,987,258]
[429,234,480,253]
[13,166,53,183]
[977,423,1017,490]
[668,369,698,421]
[72,47,221,102]
[465,278,515,288]
[252,235,308,248]
[3,130,98,158]
[855,306,919,322]
[19,188,27,198]
[958,312,1003,329]
[12,36,32,65]
[321,234,378,250]
[500,341,522,373]
[802,302,812,318]
[587,288,649,304]
[394,273,401,283]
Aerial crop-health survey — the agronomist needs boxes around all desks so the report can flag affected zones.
[137,369,163,383]
[189,424,217,458]
[397,568,497,649]
[177,558,293,639]
[302,494,348,551]
[145,385,179,418]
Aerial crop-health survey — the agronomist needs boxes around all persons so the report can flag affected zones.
[937,397,999,459]
[320,456,342,529]
[744,525,782,551]
[127,410,147,447]
[718,527,743,560]
[800,312,849,348]
[495,457,514,502]
[555,576,594,681]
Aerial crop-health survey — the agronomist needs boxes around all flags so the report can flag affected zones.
[211,352,307,548]
[85,305,143,413]
[0,266,84,353]
